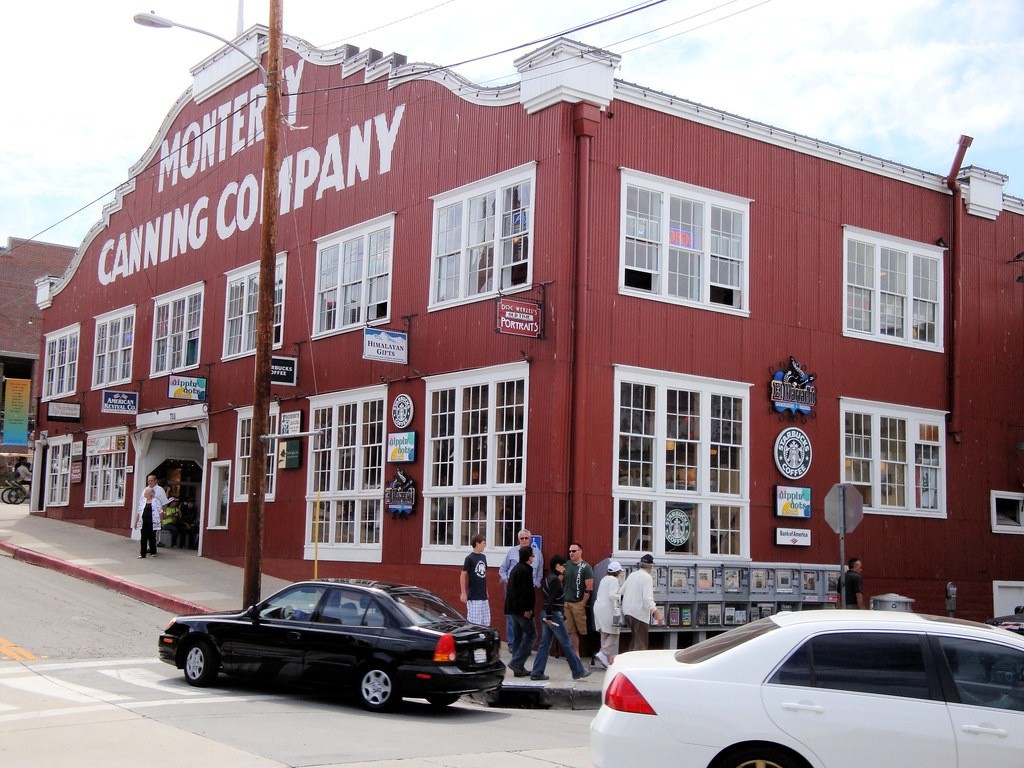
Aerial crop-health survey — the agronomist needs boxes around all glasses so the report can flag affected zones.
[519,537,530,540]
[568,550,581,553]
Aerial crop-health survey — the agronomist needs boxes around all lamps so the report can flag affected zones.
[28,316,45,325]
[666,416,675,450]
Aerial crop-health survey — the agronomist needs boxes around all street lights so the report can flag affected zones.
[129,1,285,616]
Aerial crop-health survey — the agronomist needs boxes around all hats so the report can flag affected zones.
[641,554,655,564]
[169,497,180,502]
[608,561,624,572]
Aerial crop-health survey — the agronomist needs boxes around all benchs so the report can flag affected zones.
[159,530,199,546]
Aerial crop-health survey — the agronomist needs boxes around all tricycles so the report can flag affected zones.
[1,471,30,505]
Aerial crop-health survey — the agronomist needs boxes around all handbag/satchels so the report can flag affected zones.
[612,608,628,626]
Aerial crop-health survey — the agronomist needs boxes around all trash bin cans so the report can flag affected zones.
[869,593,916,613]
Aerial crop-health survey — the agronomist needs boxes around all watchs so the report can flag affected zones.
[162,512,166,514]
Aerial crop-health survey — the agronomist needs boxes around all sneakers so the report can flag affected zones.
[595,652,610,668]
[530,675,549,680]
[573,670,591,679]
[514,671,529,677]
[151,553,156,557]
[137,556,146,559]
[507,663,532,675]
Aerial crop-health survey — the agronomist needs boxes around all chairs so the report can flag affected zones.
[340,603,360,625]
[360,596,381,624]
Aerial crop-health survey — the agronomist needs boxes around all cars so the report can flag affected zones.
[985,611,1024,639]
[586,607,1024,768]
[155,577,507,711]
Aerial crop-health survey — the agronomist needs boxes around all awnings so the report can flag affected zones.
[126,414,209,457]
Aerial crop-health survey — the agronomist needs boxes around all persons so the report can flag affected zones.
[530,554,592,681]
[834,556,866,609]
[14,456,33,496]
[562,542,593,661]
[498,528,543,653]
[460,534,491,627]
[133,475,200,558]
[593,561,625,668]
[943,642,1022,710]
[618,553,664,651]
[503,545,537,678]
